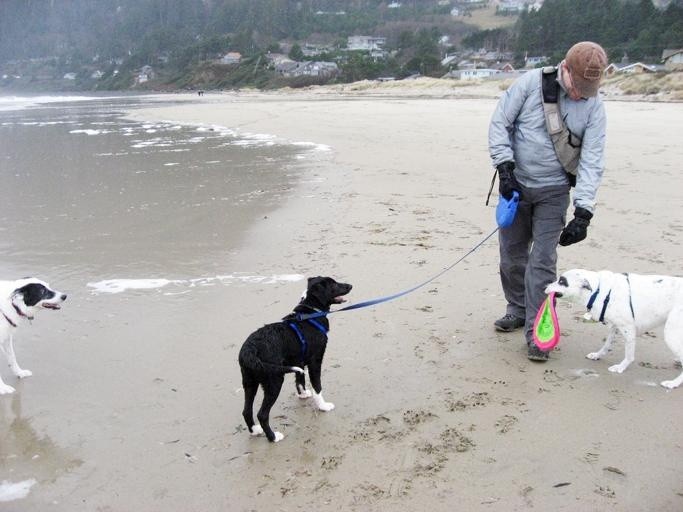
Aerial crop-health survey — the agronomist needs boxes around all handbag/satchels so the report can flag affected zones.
[550,126,581,177]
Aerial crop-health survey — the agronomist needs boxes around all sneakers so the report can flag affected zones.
[493,313,526,334]
[527,339,550,363]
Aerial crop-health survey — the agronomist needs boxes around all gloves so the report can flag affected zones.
[557,206,593,247]
[495,161,525,203]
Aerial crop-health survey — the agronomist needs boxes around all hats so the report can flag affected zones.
[565,41,608,99]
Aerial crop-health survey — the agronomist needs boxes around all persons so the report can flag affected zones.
[489,41,608,364]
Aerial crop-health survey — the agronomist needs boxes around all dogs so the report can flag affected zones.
[238,276,353,443]
[0,277,68,394]
[542,269,683,389]
